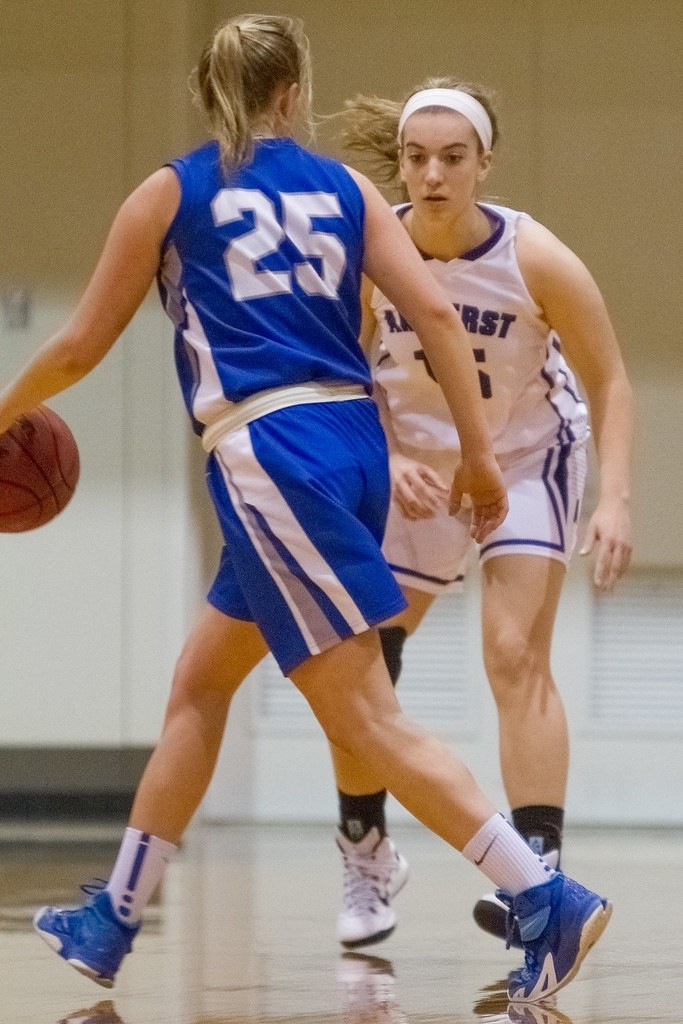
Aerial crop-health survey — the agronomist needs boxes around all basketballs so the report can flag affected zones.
[0,401,82,537]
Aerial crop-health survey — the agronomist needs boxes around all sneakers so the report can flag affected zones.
[334,826,410,948]
[473,835,561,952]
[33,877,143,988]
[495,872,613,1003]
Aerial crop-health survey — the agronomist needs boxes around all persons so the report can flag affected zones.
[328,77,635,949]
[0,14,614,1002]
